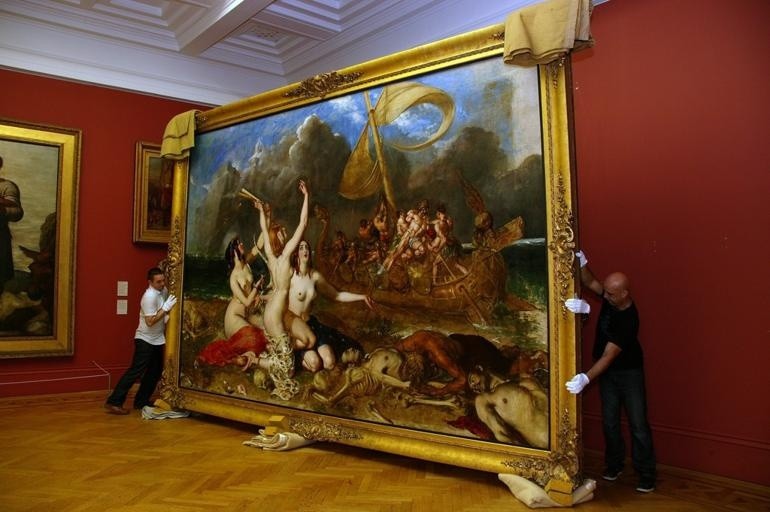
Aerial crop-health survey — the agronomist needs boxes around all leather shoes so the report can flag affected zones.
[103,401,131,416]
[133,400,155,410]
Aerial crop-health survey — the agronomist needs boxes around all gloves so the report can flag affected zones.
[566,298,592,317]
[576,250,589,268]
[162,294,178,312]
[565,373,591,396]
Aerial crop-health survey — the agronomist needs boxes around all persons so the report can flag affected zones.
[564,250,656,494]
[0,157,25,292]
[565,292,591,322]
[104,267,177,414]
[217,182,550,451]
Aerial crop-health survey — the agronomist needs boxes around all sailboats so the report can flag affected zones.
[310,82,529,326]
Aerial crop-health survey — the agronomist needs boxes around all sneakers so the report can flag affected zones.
[602,465,625,482]
[635,475,658,494]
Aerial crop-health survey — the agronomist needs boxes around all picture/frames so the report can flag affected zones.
[1,119,83,362]
[159,20,582,488]
[132,140,174,246]
